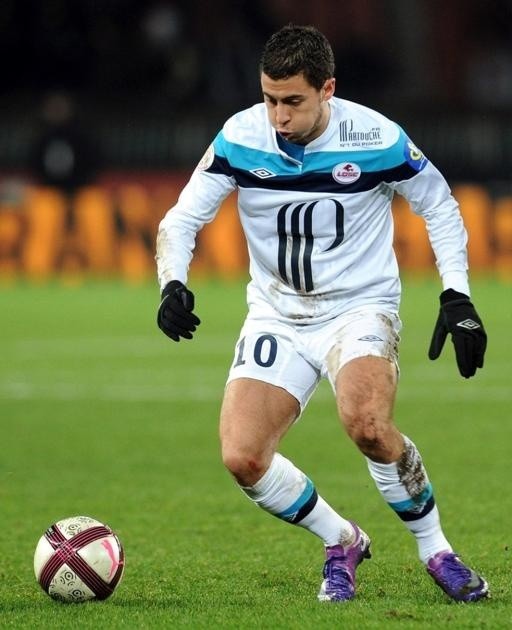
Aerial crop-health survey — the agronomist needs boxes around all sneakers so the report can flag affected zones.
[318,520,370,603]
[427,550,488,602]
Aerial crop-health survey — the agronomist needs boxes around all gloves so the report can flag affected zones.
[157,281,200,342]
[428,288,487,378]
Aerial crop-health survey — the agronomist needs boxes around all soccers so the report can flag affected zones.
[32,515,125,603]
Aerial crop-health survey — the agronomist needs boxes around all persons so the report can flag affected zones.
[391,193,437,273]
[453,181,512,281]
[154,20,494,610]
[0,168,250,281]
